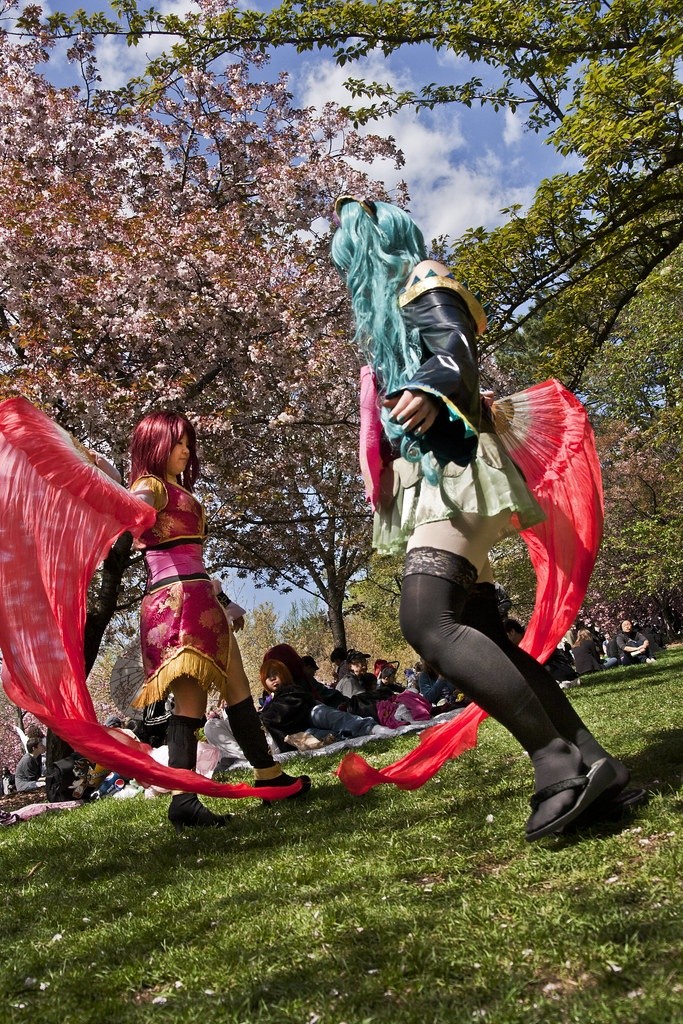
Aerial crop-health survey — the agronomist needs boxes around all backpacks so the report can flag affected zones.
[44,752,91,802]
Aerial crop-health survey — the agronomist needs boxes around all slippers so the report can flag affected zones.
[553,789,647,837]
[524,758,618,843]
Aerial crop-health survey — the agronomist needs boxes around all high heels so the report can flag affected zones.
[254,761,310,807]
[168,793,235,836]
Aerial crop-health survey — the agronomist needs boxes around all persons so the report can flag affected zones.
[0,620,654,802]
[87,411,310,826]
[331,193,647,841]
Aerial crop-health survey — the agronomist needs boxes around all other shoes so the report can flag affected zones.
[213,758,238,773]
[645,657,657,663]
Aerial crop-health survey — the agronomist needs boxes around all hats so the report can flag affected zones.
[105,717,121,727]
[302,656,319,670]
[347,651,371,662]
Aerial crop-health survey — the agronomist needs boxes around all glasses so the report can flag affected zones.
[354,658,368,664]
